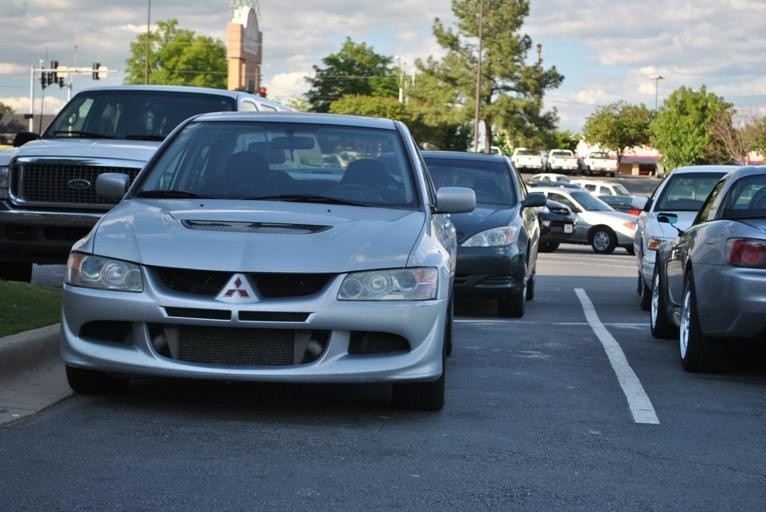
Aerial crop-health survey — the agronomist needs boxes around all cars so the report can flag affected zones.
[373,150,544,321]
[532,199,580,254]
[530,173,649,215]
[58,107,476,412]
[644,168,766,378]
[632,161,749,313]
[525,179,638,255]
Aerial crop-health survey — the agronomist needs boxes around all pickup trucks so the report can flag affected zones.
[546,149,579,174]
[580,151,618,177]
[511,146,543,173]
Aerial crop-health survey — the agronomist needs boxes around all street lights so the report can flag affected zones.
[535,42,542,80]
[649,71,665,111]
[394,54,408,104]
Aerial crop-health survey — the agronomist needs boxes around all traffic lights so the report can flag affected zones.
[256,86,268,98]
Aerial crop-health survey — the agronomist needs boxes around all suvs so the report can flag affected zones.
[0,82,287,285]
